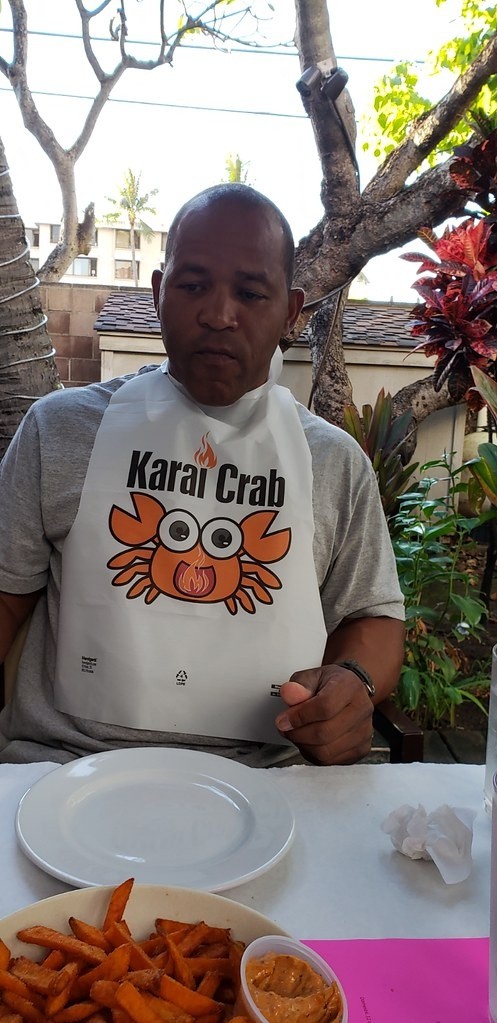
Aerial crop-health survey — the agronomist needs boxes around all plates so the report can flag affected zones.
[15,747,296,893]
[0,884,300,1023]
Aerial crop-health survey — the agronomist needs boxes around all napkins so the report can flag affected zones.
[380,802,477,885]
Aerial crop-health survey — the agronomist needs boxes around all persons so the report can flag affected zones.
[0,183,406,769]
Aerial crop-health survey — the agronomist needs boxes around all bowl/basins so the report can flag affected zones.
[233,935,349,1023]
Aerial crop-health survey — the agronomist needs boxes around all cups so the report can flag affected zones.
[484,644,497,811]
[490,773,497,1023]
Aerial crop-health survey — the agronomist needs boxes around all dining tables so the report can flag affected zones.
[0,759,497,1023]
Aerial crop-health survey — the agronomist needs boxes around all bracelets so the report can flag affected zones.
[336,658,376,697]
